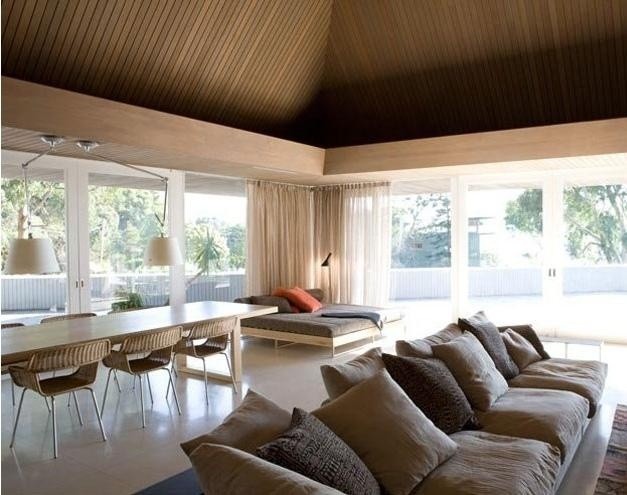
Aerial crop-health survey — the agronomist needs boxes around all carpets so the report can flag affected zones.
[595,403,627,495]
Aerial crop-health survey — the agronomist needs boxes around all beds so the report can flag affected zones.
[234,289,404,359]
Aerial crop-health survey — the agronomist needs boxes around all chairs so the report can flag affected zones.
[1,305,237,459]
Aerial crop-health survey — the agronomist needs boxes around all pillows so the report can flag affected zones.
[250,287,325,315]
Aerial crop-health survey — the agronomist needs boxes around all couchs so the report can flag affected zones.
[180,358,608,495]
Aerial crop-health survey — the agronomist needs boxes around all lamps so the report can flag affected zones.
[76,140,183,267]
[321,253,332,302]
[5,136,66,275]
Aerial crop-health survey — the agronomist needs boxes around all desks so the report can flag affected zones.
[1,302,278,384]
